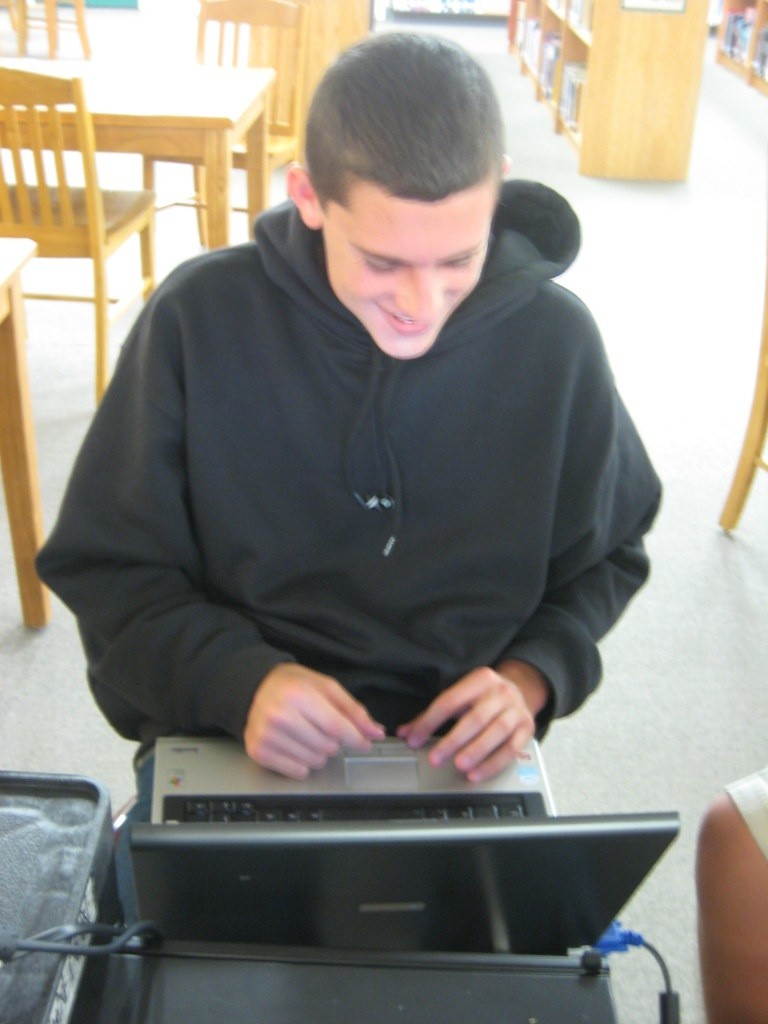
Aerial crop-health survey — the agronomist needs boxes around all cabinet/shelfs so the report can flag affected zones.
[505,0,711,186]
[717,0,768,96]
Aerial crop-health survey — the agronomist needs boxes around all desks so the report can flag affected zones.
[0,60,280,254]
[0,277,51,634]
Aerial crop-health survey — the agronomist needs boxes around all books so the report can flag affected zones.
[511,0,587,131]
[722,2,767,81]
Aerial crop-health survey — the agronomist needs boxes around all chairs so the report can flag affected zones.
[0,0,319,414]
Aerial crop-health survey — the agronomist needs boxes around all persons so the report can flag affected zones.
[35,31,662,926]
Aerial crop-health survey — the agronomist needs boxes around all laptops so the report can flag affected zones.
[128,736,682,956]
